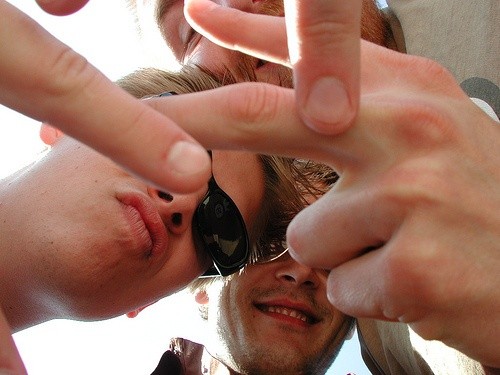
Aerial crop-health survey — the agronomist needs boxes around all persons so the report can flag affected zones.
[148,0,407,166]
[0,0,322,375]
[137,1,498,375]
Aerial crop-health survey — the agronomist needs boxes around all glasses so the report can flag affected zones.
[252,223,289,265]
[141,91,252,279]
